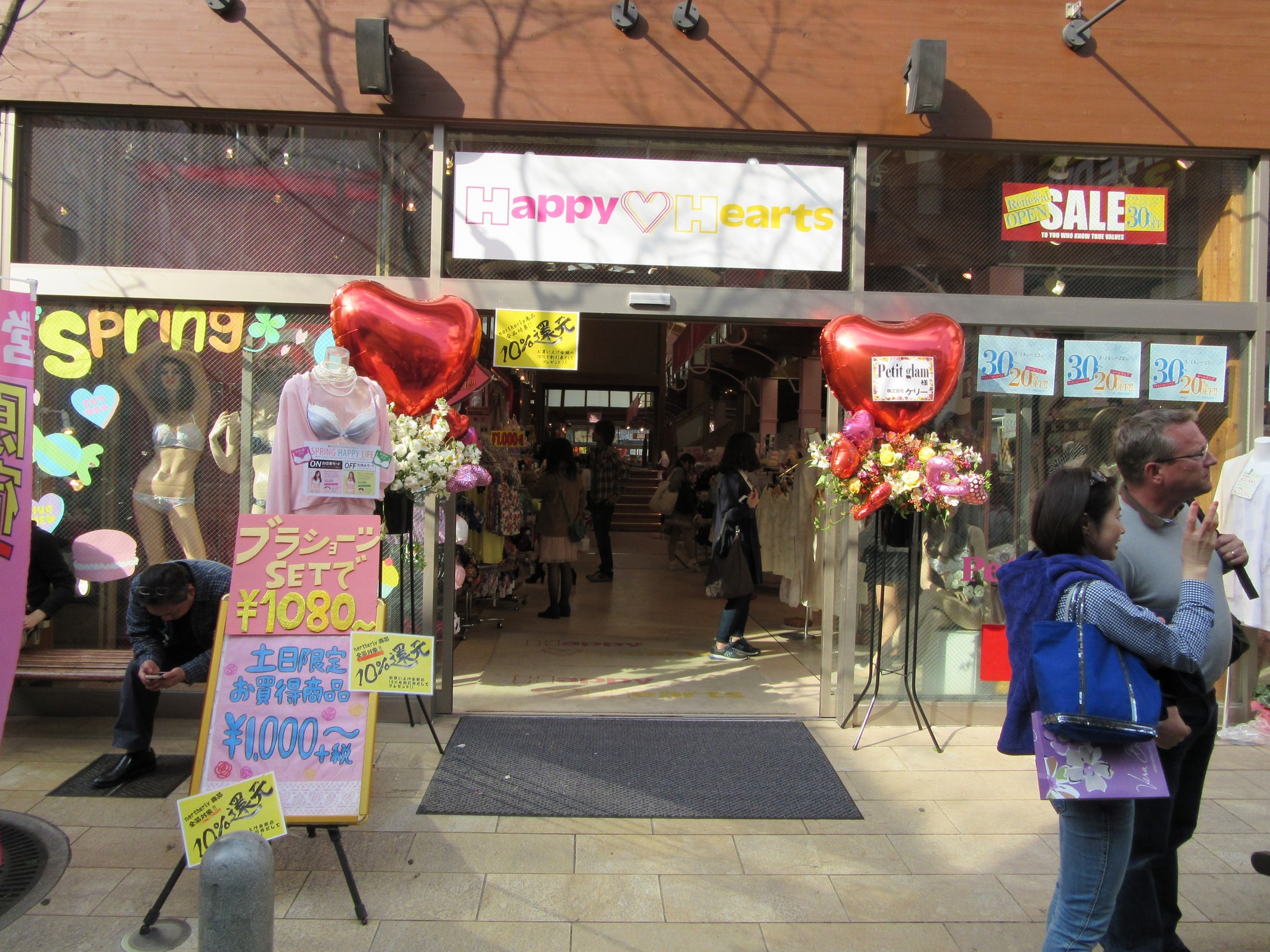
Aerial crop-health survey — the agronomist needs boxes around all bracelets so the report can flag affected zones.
[615,493,620,497]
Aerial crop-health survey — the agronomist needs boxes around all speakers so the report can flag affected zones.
[354,18,396,96]
[903,37,947,115]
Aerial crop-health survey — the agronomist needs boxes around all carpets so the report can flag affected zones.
[417,714,863,820]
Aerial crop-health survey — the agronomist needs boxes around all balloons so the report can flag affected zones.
[818,310,990,521]
[327,280,492,493]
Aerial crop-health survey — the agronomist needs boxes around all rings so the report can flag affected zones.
[1230,551,1237,558]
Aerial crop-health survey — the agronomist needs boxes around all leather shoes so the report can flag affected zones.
[91,746,157,788]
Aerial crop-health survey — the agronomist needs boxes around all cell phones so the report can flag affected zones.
[144,671,167,679]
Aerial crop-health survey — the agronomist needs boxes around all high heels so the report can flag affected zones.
[525,569,545,584]
[571,568,577,586]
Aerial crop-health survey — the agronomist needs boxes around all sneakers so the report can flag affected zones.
[669,564,685,571]
[687,562,701,572]
[538,606,560,619]
[560,602,571,616]
[708,643,747,660]
[729,636,761,656]
[585,570,613,582]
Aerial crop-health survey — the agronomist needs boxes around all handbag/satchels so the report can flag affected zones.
[569,520,586,543]
[1030,709,1171,800]
[1032,573,1161,740]
[705,511,754,598]
[649,467,685,516]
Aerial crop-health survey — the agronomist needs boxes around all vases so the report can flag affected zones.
[879,505,916,549]
[382,489,414,533]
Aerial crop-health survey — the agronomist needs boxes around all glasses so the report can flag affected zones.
[136,585,172,596]
[1088,467,1108,486]
[1161,443,1208,461]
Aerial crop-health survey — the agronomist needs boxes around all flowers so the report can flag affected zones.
[388,398,483,502]
[805,429,992,533]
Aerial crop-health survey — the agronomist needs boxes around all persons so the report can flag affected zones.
[345,471,356,494]
[115,335,298,570]
[656,450,670,486]
[857,403,1127,684]
[310,470,322,494]
[584,420,631,582]
[519,437,594,619]
[993,465,1218,951]
[1096,405,1252,951]
[266,344,396,515]
[92,560,233,790]
[660,432,917,674]
[17,520,77,651]
